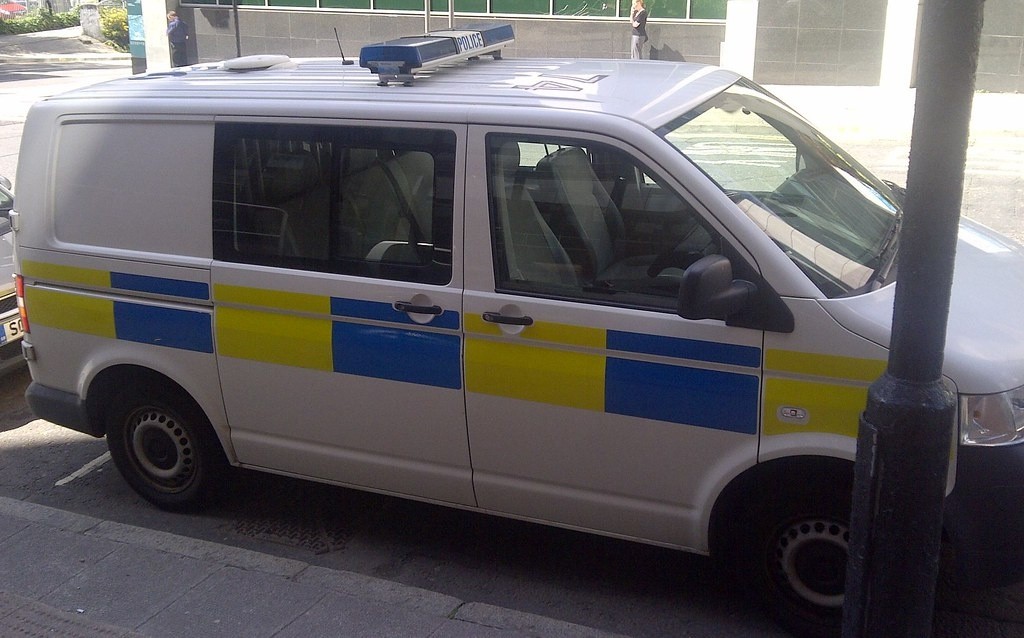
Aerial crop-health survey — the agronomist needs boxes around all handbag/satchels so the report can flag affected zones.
[643,32,648,42]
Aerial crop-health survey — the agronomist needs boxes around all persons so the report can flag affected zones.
[630,0,648,60]
[167,11,190,68]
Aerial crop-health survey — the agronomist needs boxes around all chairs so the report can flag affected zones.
[492,139,581,288]
[378,148,434,243]
[343,149,426,266]
[536,144,685,297]
[253,150,371,261]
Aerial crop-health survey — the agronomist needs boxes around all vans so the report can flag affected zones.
[10,21,1023,629]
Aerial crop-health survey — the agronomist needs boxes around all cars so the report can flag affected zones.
[0,0,28,22]
[0,175,25,376]
